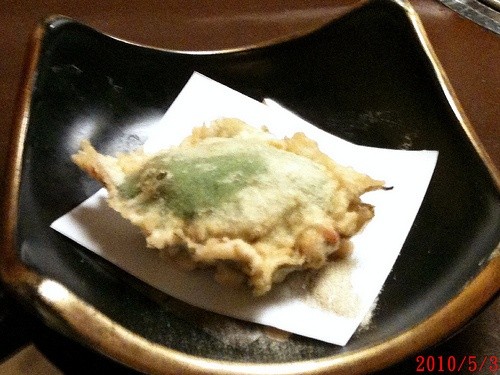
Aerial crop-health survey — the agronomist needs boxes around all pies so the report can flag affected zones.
[72,115,391,300]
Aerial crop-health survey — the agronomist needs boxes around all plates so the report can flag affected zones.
[1,0,500,375]
